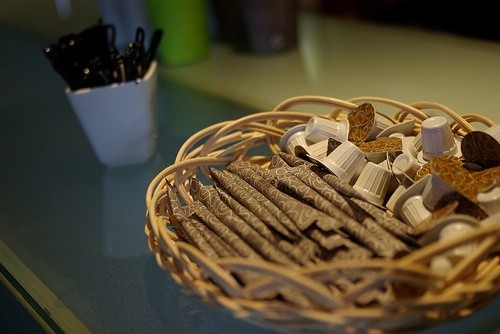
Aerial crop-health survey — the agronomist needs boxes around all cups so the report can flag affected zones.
[65,61,158,167]
[145,0,299,66]
